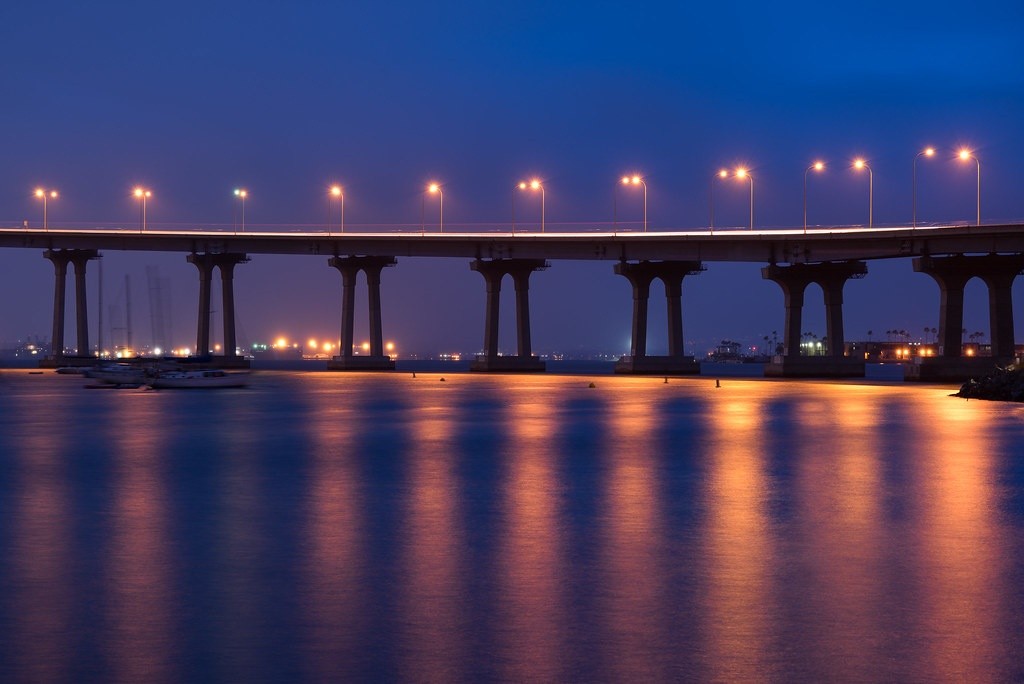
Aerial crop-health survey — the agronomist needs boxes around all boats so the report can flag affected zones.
[55,366,159,383]
[136,371,256,387]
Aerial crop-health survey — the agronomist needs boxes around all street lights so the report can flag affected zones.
[734,168,753,229]
[241,189,247,231]
[614,176,629,232]
[711,169,727,231]
[35,188,57,229]
[134,189,152,231]
[913,148,936,227]
[332,187,343,233]
[633,176,647,230]
[429,184,443,232]
[853,159,873,227]
[512,183,525,233]
[234,190,239,230]
[531,180,545,232]
[804,163,825,229]
[959,150,980,225]
